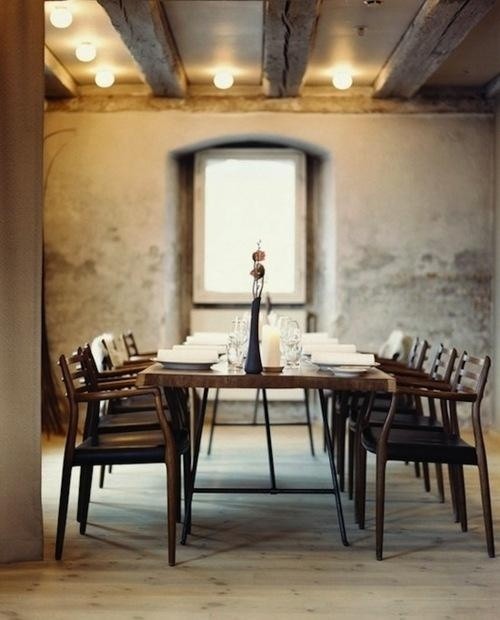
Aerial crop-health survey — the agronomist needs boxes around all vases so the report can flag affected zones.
[244,297,263,374]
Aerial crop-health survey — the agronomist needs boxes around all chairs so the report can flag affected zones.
[319,333,494,562]
[53,330,203,568]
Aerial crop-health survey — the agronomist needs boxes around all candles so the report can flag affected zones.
[262,324,281,366]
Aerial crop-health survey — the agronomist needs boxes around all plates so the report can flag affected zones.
[305,360,381,371]
[328,367,367,377]
[149,358,219,370]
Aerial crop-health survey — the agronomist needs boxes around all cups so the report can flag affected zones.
[227,311,302,370]
